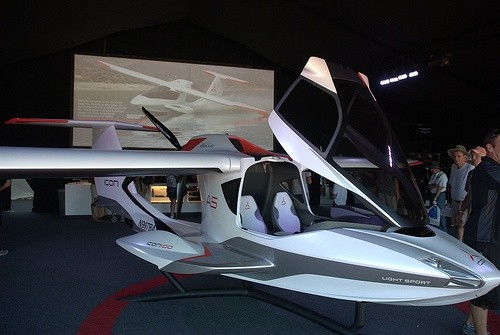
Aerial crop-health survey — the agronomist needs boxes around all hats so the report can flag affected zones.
[447,145,472,161]
[426,160,442,171]
[470,147,487,157]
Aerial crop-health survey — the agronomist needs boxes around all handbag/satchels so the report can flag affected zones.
[427,201,440,226]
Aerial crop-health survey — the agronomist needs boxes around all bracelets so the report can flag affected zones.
[434,198,436,200]
[458,211,463,213]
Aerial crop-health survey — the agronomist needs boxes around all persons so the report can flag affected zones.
[287,171,312,204]
[457,146,486,244]
[167,175,179,217]
[0,182,10,255]
[331,183,348,207]
[111,211,125,222]
[427,161,449,232]
[462,129,500,335]
[365,169,375,191]
[376,169,400,213]
[447,145,476,241]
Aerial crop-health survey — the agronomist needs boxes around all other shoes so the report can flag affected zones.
[0,250,8,256]
[170,214,174,219]
[120,216,124,222]
[112,217,117,222]
[460,323,477,335]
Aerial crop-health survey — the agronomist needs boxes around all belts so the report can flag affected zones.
[433,192,445,196]
[454,200,463,203]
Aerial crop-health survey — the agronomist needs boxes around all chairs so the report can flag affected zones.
[241,195,269,233]
[274,191,301,233]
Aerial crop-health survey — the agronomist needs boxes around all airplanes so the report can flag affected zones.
[0,54,500,335]
